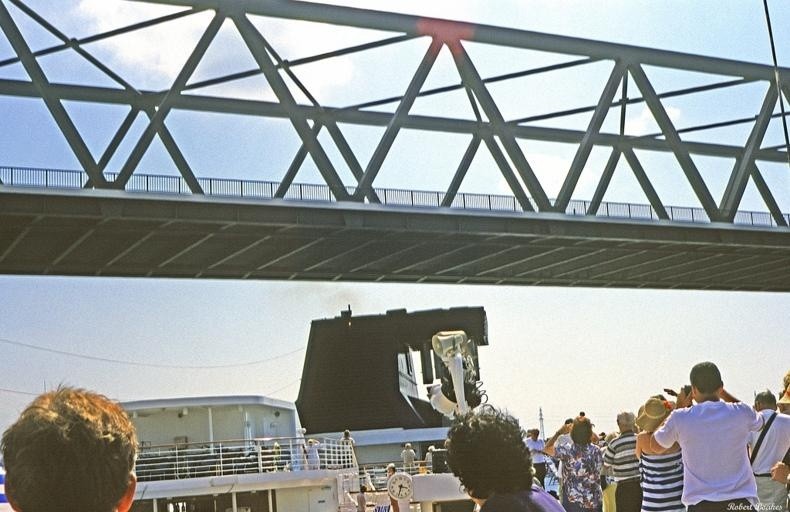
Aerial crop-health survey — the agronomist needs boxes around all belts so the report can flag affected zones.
[752,472,771,478]
[617,475,642,486]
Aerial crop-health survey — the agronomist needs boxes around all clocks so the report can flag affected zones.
[387,472,413,501]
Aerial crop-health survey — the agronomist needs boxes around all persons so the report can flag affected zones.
[385,462,400,511]
[425,444,436,467]
[339,429,355,448]
[521,375,790,510]
[442,401,569,511]
[649,360,767,512]
[355,485,368,511]
[303,438,321,470]
[400,442,417,470]
[1,382,139,511]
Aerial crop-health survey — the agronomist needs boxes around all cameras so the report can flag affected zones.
[683,385,692,395]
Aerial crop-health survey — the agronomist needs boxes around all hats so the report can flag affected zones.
[384,462,395,470]
[635,396,677,434]
[775,383,790,405]
